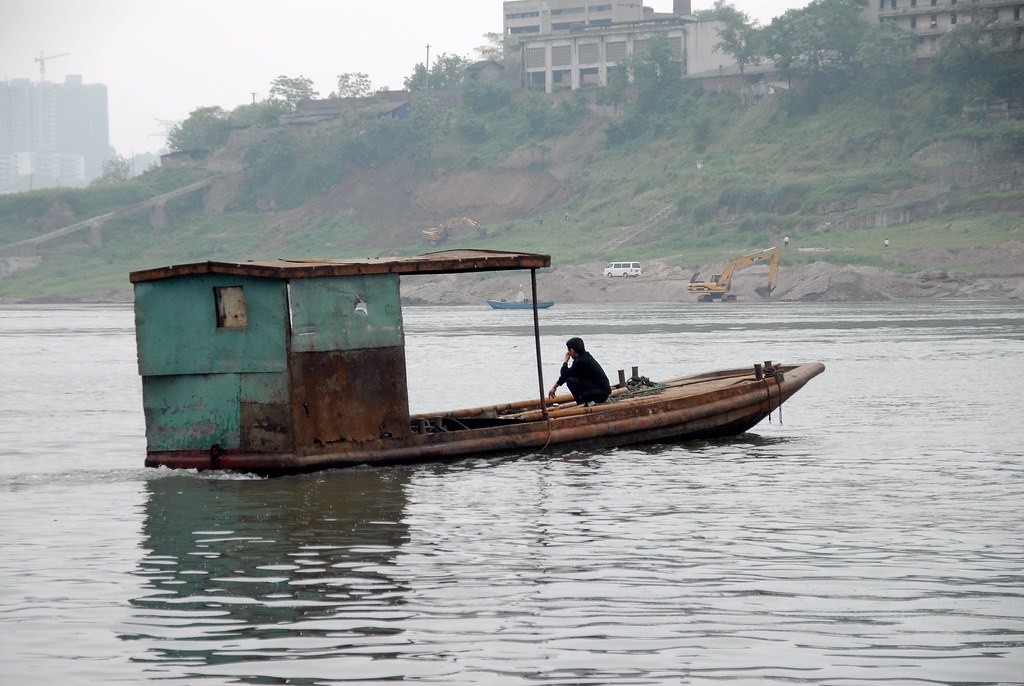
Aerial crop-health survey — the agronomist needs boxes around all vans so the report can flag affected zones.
[603,261,642,278]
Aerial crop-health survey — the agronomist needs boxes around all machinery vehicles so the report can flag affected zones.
[686,246,781,303]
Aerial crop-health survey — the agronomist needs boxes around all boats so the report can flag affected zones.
[486,299,555,310]
[128,249,826,478]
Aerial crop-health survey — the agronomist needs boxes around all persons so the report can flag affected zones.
[549,337,611,408]
